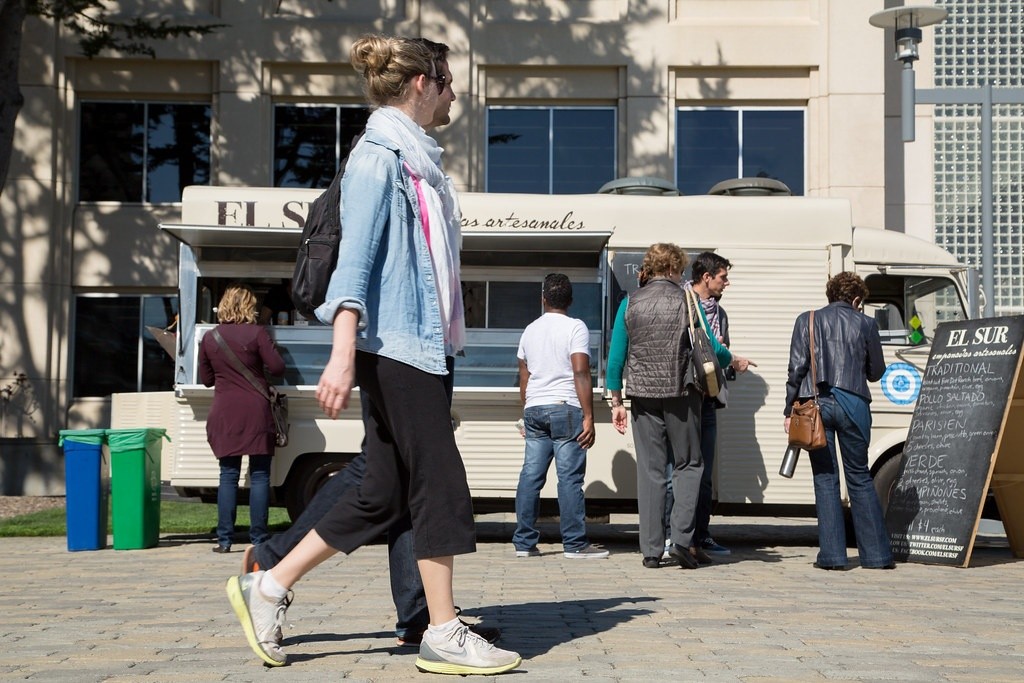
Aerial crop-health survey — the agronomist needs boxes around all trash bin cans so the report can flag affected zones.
[60,429,108,552]
[110,429,167,550]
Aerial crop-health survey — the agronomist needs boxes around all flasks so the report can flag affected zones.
[779,443,801,478]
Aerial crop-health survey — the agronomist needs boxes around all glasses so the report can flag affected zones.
[419,73,447,96]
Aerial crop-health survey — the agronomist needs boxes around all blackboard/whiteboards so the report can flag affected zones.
[888,316,1024,567]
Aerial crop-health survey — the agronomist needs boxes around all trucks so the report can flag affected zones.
[155,174,980,539]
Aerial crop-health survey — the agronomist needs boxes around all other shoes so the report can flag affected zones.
[213,545,230,553]
[814,561,845,570]
[641,557,659,567]
[669,542,698,569]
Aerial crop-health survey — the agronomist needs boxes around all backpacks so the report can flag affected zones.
[290,127,369,323]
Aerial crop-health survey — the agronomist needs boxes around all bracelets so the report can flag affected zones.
[611,399,623,407]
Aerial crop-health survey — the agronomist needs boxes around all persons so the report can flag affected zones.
[199,286,286,554]
[784,270,898,571]
[224,34,524,676]
[511,273,609,559]
[605,242,758,570]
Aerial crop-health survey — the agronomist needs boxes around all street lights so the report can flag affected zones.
[869,5,953,232]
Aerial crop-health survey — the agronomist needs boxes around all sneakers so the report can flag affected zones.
[396,606,501,645]
[662,539,671,559]
[414,623,521,674]
[693,537,730,554]
[224,570,295,666]
[563,544,610,559]
[516,547,539,557]
[689,545,712,562]
[242,545,259,575]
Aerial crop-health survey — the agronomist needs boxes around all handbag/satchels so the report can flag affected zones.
[789,399,827,450]
[269,388,291,448]
[683,283,729,410]
